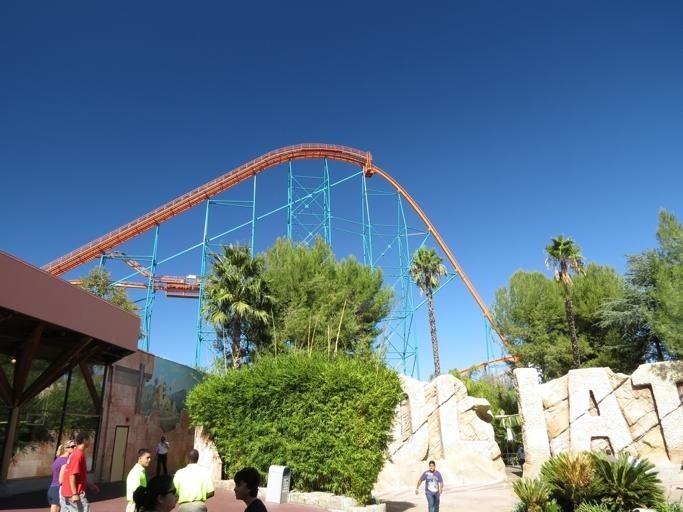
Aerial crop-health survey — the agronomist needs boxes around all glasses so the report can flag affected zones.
[66,445,75,448]
[165,488,176,494]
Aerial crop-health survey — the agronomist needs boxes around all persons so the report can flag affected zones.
[518,443,527,471]
[415,460,443,512]
[234,467,268,512]
[173,448,215,512]
[130,475,178,512]
[59,431,93,512]
[125,448,152,512]
[46,440,76,512]
[155,436,170,476]
